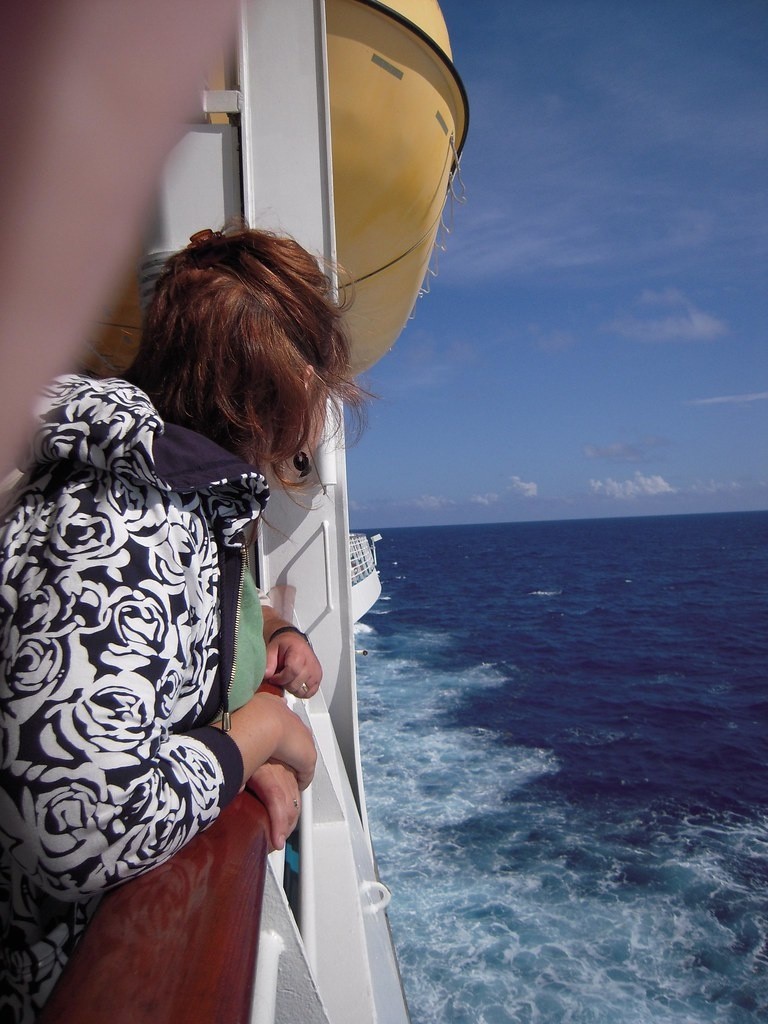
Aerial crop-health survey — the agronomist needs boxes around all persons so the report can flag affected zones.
[0,0,385,1024]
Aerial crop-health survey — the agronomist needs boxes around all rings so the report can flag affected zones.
[294,800,298,806]
[302,682,309,693]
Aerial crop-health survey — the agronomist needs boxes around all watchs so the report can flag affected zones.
[268,627,312,649]
